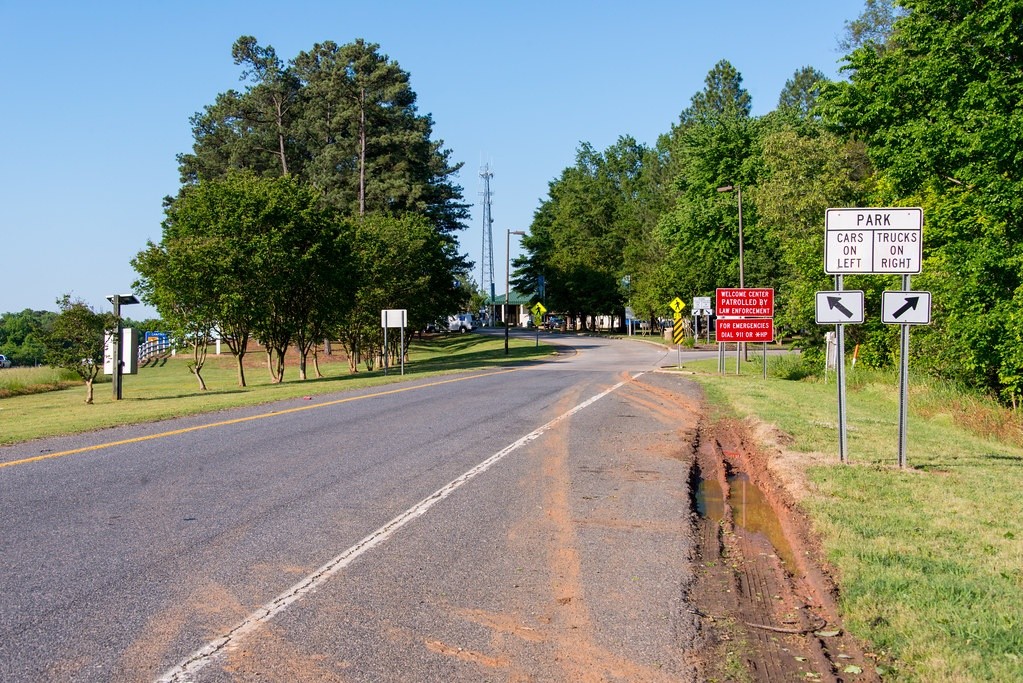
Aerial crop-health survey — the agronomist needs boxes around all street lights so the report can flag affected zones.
[716,182,748,360]
[504,228,524,353]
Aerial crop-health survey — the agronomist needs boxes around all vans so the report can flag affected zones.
[425,313,479,333]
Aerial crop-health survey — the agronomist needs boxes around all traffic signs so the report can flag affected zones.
[824,207,923,274]
[690,297,712,315]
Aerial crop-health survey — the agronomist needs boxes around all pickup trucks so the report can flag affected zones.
[0,354,12,368]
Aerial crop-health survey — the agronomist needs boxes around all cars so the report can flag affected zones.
[550,318,566,327]
